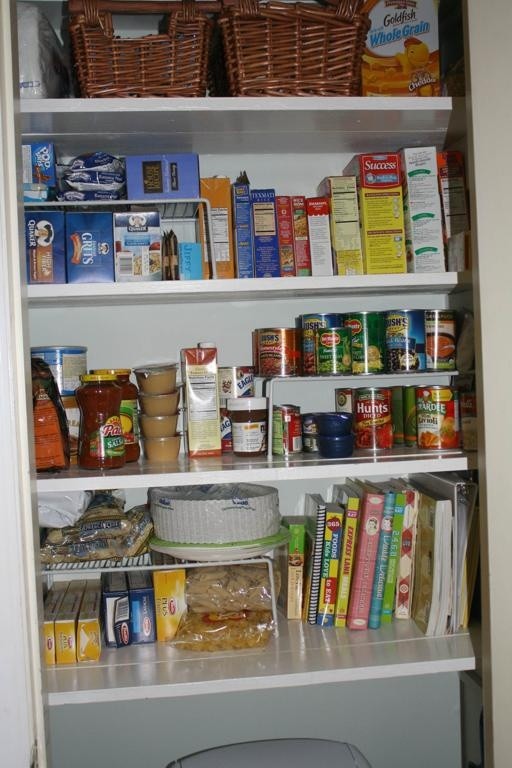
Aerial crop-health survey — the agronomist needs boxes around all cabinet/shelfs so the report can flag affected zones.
[1,0,495,768]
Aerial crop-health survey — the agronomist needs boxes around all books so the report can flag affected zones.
[283,471,481,638]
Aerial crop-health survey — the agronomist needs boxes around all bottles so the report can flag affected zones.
[386,309,426,374]
[226,397,269,456]
[29,345,86,395]
[74,374,126,469]
[220,408,233,453]
[346,312,388,375]
[424,309,458,372]
[300,313,345,374]
[257,327,300,376]
[57,397,81,466]
[217,366,255,409]
[459,392,478,451]
[90,368,140,462]
[354,387,393,450]
[315,328,353,375]
[335,388,355,414]
[415,384,458,450]
[403,385,431,448]
[282,404,303,453]
[390,385,405,444]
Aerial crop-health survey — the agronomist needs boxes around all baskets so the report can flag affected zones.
[64,0,223,98]
[217,0,373,96]
